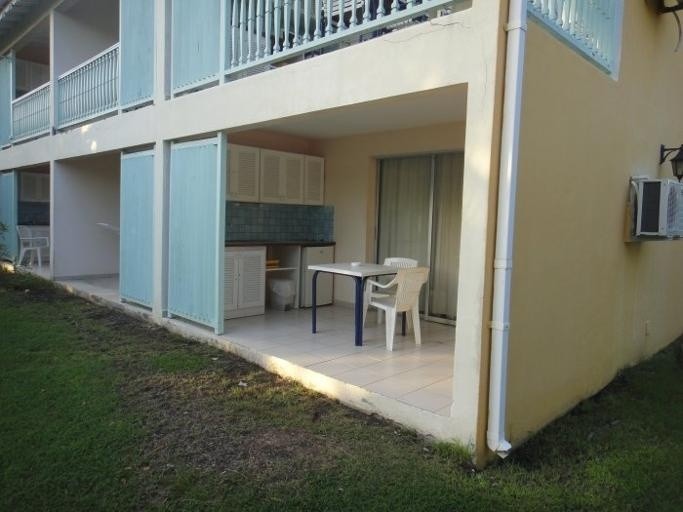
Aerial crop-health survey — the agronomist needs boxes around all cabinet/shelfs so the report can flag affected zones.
[302,153,326,206]
[220,239,267,324]
[226,143,258,205]
[265,244,302,312]
[259,149,304,207]
[17,171,49,205]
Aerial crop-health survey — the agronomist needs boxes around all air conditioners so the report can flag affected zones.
[622,174,683,242]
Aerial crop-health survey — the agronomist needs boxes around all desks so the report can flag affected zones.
[305,259,411,346]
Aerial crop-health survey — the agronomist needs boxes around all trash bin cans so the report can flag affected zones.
[269,278,296,311]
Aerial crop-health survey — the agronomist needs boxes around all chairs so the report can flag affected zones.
[361,266,433,354]
[12,222,50,272]
[361,254,422,335]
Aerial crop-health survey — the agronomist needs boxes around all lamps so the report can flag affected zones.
[659,138,683,184]
